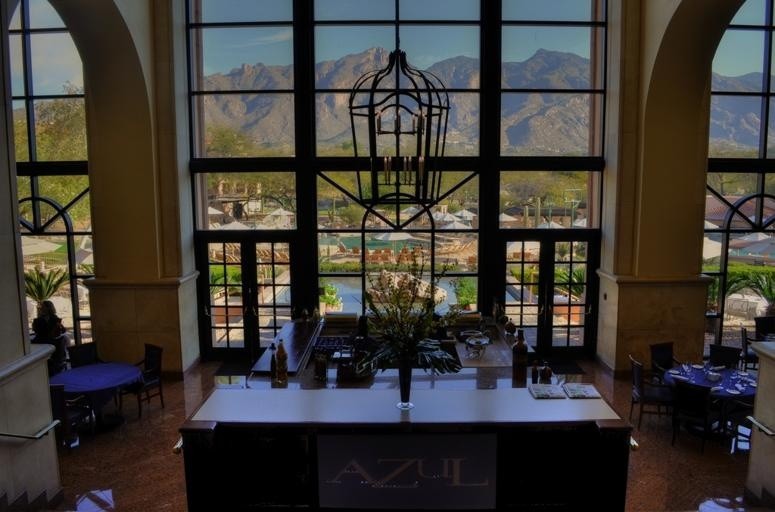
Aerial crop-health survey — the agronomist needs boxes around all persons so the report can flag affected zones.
[32,300,67,346]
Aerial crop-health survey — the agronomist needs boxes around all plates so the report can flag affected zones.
[669,369,680,374]
[737,370,749,376]
[749,382,757,387]
[693,363,704,369]
[726,389,741,395]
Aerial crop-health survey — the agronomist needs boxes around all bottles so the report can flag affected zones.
[268,339,289,387]
[511,329,529,387]
[531,361,553,382]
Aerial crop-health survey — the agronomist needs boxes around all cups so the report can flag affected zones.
[461,329,490,360]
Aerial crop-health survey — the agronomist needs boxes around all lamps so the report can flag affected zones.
[349,0,451,205]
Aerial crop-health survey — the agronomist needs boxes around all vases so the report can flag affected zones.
[395,353,415,411]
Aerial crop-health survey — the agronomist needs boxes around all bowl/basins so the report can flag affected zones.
[707,372,721,381]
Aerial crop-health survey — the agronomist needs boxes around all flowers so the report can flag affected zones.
[352,244,464,376]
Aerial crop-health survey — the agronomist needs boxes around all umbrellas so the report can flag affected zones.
[702,236,733,260]
[705,219,720,229]
[399,206,586,229]
[207,206,297,230]
[374,233,413,257]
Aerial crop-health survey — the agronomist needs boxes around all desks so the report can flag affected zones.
[176,382,635,512]
[49,362,145,432]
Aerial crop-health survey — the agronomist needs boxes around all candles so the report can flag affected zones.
[375,109,427,185]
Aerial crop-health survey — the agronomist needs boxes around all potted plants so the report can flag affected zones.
[23,265,70,370]
[703,268,762,332]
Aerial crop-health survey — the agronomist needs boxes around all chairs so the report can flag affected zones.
[50,384,93,436]
[629,314,775,459]
[118,342,165,418]
[67,342,118,409]
